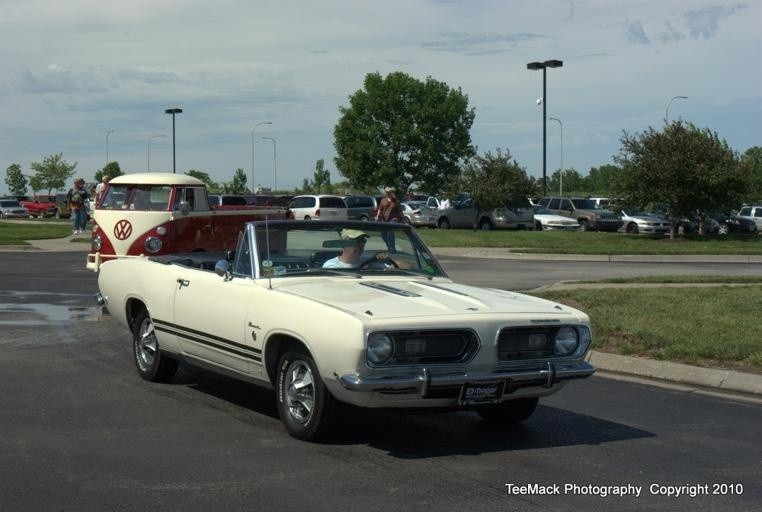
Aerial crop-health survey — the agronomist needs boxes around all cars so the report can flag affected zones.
[1,192,136,219]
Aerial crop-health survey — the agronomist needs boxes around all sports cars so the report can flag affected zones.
[97,219,596,444]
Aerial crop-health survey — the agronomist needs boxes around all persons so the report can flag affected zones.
[319,227,393,271]
[371,187,406,253]
[65,177,92,237]
[95,174,117,209]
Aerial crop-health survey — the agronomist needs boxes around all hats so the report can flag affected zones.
[340,228,370,240]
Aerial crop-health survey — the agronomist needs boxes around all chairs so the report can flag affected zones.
[200,249,343,277]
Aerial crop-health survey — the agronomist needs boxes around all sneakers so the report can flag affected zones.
[79,227,83,234]
[74,229,78,235]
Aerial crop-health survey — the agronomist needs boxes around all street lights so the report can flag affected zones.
[106,130,114,165]
[524,59,563,196]
[147,134,167,173]
[665,94,690,123]
[250,122,273,194]
[165,109,183,174]
[547,116,563,196]
[261,137,277,193]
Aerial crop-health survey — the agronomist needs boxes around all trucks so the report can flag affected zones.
[87,173,292,273]
